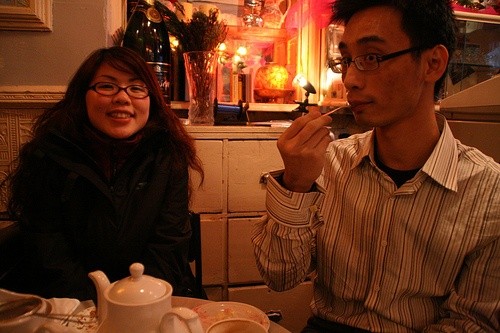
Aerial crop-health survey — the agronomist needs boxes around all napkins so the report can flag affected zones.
[50,298,99,333]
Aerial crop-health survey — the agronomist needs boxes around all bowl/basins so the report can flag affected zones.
[0,289,47,333]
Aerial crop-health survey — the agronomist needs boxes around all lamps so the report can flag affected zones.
[253,59,294,104]
[291,75,317,119]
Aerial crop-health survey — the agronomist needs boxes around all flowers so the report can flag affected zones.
[154,1,229,53]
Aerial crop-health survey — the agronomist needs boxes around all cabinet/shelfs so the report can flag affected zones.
[183,0,500,333]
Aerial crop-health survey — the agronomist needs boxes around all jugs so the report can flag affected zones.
[88,262,204,333]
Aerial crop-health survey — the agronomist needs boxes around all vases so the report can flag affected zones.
[182,50,220,125]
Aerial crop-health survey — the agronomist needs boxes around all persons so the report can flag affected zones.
[7,47,209,301]
[250,0,500,333]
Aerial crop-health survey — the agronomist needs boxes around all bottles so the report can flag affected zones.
[123,0,174,107]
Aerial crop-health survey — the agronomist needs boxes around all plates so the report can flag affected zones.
[68,305,98,333]
[43,299,54,322]
[190,301,270,331]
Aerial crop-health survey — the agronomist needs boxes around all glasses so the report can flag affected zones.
[87,81,150,99]
[327,46,413,74]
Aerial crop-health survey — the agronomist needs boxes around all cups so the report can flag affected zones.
[205,318,267,333]
